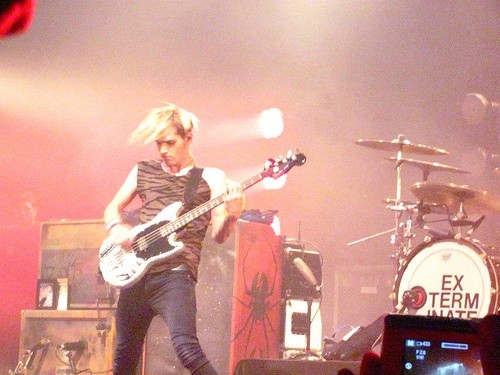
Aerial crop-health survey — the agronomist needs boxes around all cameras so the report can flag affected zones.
[379,314,485,375]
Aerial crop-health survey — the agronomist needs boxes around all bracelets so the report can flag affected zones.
[104,219,124,234]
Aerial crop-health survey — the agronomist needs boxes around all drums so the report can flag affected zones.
[391,235,500,319]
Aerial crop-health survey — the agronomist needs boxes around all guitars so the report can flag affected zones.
[99,147,307,289]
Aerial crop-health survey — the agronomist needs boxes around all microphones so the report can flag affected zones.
[57,341,84,351]
[467,215,485,235]
[294,258,321,294]
[25,338,49,354]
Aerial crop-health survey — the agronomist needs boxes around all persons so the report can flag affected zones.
[103,101,243,375]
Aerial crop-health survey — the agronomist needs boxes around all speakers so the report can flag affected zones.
[142,220,283,375]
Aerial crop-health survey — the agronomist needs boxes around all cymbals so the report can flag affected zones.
[382,197,418,210]
[353,139,451,158]
[384,155,474,176]
[409,180,500,214]
